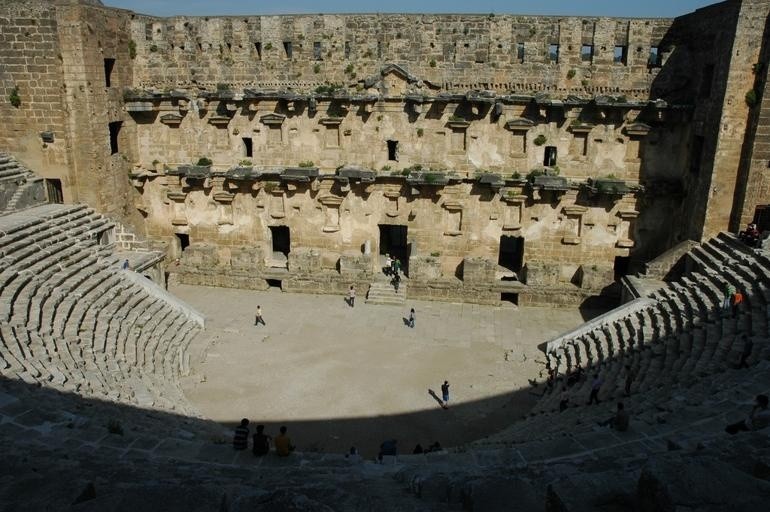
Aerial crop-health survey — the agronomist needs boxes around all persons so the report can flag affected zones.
[347,286,355,307]
[123,259,129,270]
[725,395,770,435]
[255,306,266,325]
[345,437,442,464]
[441,381,449,405]
[385,252,403,293]
[546,363,635,431]
[233,419,296,457]
[410,308,415,327]
